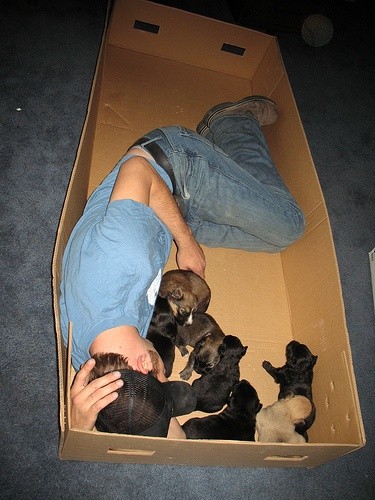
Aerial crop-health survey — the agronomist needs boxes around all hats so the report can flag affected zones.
[93,369,198,439]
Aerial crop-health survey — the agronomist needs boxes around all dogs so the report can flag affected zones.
[182,379,263,442]
[146,297,226,381]
[254,390,314,443]
[192,335,248,413]
[262,340,318,434]
[158,269,211,325]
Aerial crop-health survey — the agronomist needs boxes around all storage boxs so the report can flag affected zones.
[52,0,366,469]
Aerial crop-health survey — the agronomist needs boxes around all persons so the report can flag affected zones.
[60,96,305,437]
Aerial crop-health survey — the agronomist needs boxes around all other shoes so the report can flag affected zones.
[196,95,279,136]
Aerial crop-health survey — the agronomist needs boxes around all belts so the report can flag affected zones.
[127,138,177,195]
